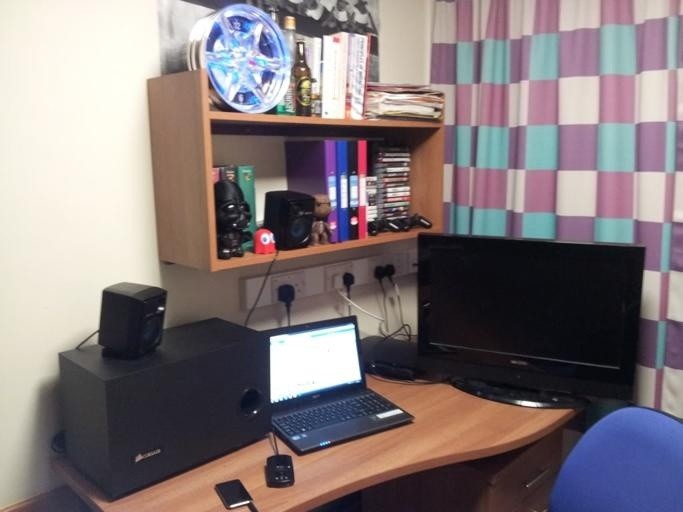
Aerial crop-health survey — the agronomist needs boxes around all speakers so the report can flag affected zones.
[59,317,270,501]
[97,281,169,357]
[264,190,315,251]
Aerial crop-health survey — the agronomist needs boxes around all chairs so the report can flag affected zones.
[548,403,683,512]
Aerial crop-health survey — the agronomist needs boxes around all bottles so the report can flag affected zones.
[292,42,312,117]
[278,16,297,114]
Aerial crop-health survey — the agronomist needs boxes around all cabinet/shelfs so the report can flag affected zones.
[148,68,445,273]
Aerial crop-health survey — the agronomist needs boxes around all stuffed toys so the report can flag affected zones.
[254,227,277,256]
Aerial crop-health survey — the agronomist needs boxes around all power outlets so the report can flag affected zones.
[241,250,419,310]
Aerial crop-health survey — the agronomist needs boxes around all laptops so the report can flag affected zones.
[262,314,416,456]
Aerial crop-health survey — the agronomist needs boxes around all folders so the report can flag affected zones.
[285,140,368,244]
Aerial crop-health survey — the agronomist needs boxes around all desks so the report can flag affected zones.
[53,361,588,512]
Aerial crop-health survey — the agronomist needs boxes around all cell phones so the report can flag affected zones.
[214,479,258,512]
[263,454,296,488]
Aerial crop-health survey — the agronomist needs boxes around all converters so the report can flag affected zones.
[278,284,295,303]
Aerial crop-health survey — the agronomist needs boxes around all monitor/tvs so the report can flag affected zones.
[415,230,647,410]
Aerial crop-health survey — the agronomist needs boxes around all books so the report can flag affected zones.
[235,163,257,255]
[284,135,415,245]
[212,167,221,186]
[304,32,444,121]
[213,162,238,186]
